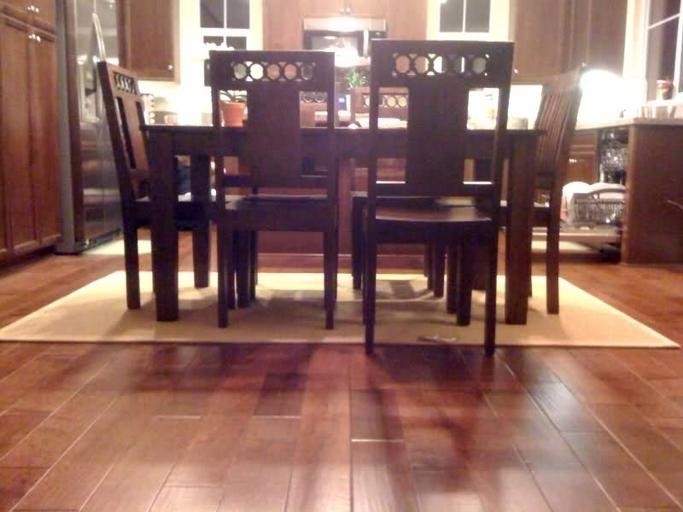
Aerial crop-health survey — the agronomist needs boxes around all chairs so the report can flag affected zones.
[208,50,336,331]
[98,63,247,309]
[360,40,516,356]
[450,64,586,316]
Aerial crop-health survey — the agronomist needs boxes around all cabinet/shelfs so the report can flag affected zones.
[145,119,683,264]
[0,0,60,263]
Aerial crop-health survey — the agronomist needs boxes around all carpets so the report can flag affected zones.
[0,269,683,350]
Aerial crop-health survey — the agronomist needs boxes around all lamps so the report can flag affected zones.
[324,38,362,68]
